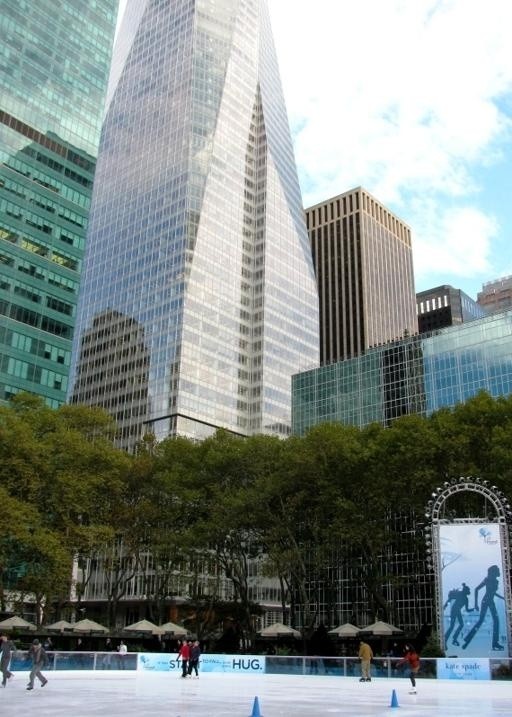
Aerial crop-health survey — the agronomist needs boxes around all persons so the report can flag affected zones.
[74,639,84,667]
[174,639,201,679]
[0,635,17,688]
[462,565,504,649]
[116,640,128,670]
[395,643,421,693]
[102,638,113,670]
[358,641,373,682]
[443,583,476,650]
[24,638,49,690]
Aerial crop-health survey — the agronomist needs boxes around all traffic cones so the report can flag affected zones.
[249,695,267,717]
[388,687,402,710]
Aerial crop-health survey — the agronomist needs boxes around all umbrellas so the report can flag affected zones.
[64,619,110,637]
[0,615,38,639]
[123,619,165,638]
[46,620,73,637]
[159,621,192,639]
[327,623,361,638]
[255,623,301,640]
[359,621,403,649]
